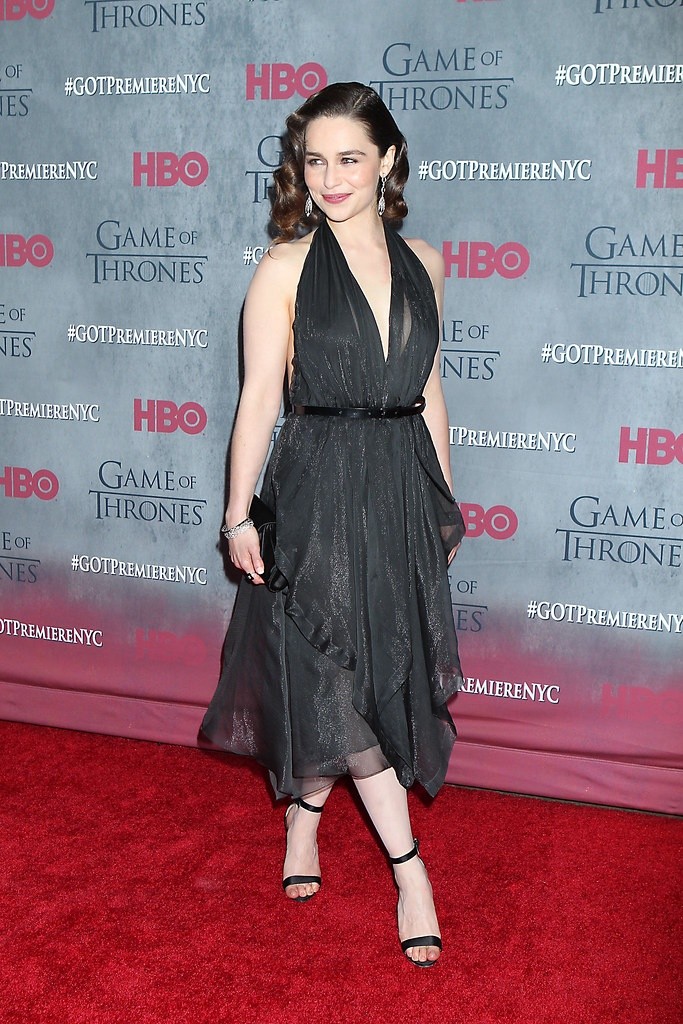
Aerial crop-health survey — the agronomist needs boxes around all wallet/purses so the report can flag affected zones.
[247,495,294,593]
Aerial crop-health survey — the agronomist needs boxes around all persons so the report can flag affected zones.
[197,81,467,967]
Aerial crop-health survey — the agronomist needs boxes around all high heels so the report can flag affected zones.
[282,796,324,902]
[388,838,444,968]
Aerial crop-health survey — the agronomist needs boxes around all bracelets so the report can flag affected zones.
[220,517,254,539]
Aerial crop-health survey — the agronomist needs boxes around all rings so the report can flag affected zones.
[247,573,257,580]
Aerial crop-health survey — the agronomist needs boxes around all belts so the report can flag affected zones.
[287,397,426,420]
